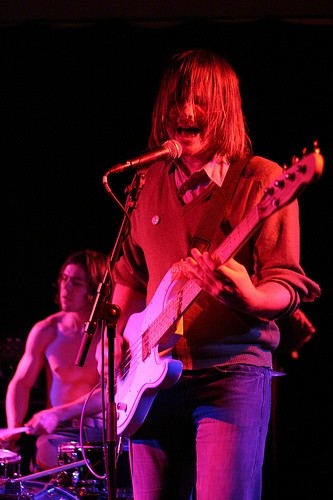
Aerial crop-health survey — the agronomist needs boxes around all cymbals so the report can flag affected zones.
[0,426,29,443]
[0,447,21,465]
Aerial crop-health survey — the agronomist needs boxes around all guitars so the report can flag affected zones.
[102,140,325,437]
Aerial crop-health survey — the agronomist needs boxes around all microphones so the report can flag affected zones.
[106,139,182,176]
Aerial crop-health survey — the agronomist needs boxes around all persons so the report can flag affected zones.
[6,250,112,477]
[95,46,302,500]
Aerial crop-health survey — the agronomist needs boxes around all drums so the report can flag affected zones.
[55,440,133,500]
[0,477,81,499]
